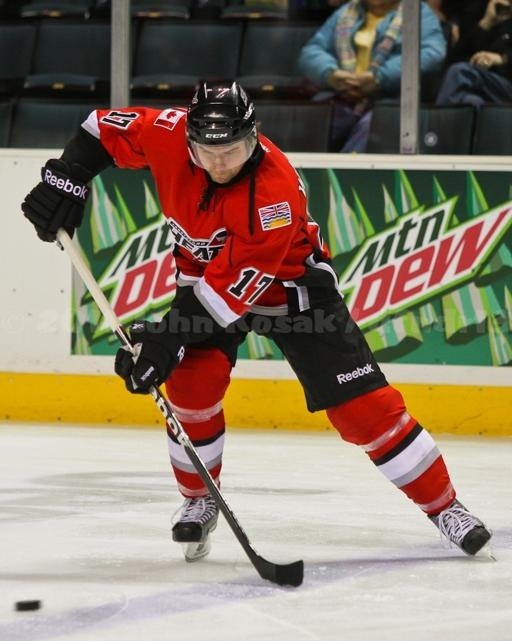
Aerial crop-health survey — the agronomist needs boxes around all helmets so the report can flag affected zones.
[184,77,259,173]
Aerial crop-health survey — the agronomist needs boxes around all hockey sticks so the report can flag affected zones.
[54,226,304,590]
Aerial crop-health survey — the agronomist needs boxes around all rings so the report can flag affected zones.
[481,60,488,66]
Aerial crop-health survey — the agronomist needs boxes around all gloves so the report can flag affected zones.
[19,150,97,251]
[113,318,187,395]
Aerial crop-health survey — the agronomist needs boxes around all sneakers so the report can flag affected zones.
[427,498,493,557]
[170,480,220,543]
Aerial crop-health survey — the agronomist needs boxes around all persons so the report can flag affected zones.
[294,1,447,155]
[434,0,511,108]
[18,79,497,565]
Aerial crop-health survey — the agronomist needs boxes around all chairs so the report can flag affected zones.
[1,0,511,154]
